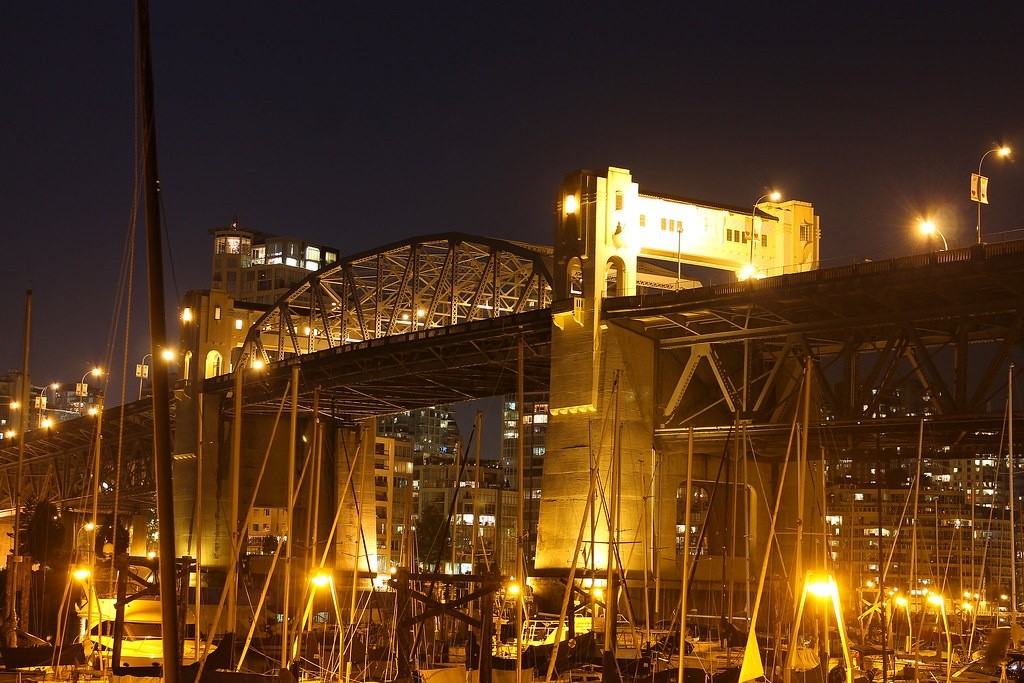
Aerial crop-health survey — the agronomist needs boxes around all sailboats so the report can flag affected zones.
[1,5,1024,682]
